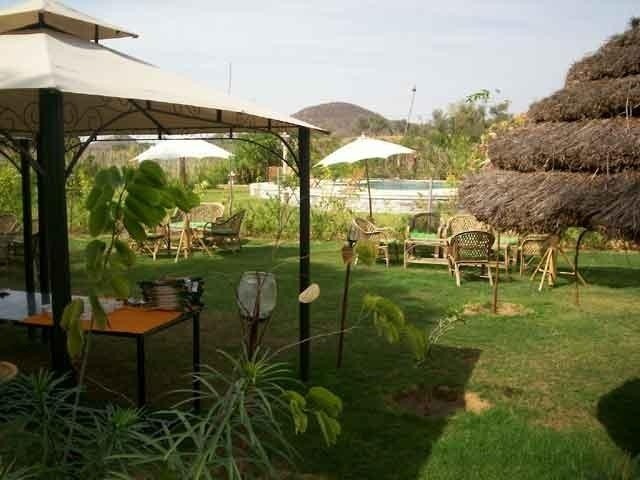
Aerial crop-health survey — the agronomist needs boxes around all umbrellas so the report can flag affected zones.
[309,136,416,217]
[124,136,237,201]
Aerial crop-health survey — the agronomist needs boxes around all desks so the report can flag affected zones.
[26,295,203,420]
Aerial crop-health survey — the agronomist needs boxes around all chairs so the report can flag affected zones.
[344,210,562,288]
[0,212,40,263]
[123,203,247,262]
